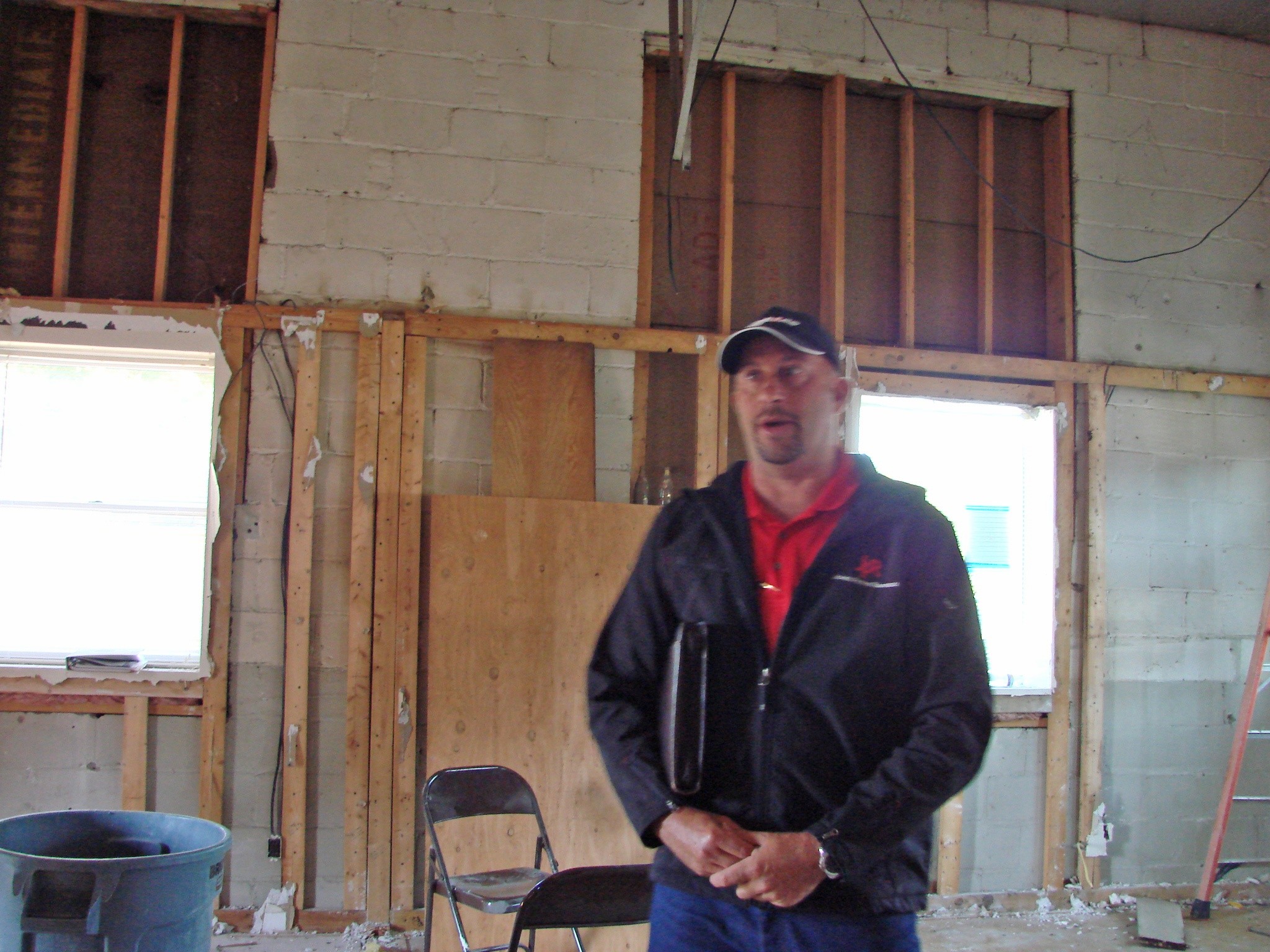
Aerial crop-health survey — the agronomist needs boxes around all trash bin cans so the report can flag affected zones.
[0,809,233,952]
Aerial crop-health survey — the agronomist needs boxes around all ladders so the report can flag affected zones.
[1184,571,1269,919]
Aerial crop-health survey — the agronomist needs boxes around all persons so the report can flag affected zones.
[586,305,994,950]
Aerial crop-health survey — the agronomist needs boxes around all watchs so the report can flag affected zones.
[818,847,842,880]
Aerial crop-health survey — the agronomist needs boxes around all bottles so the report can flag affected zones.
[634,465,649,504]
[658,466,673,505]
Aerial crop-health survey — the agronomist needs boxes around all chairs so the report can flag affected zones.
[422,765,586,952]
[508,863,655,952]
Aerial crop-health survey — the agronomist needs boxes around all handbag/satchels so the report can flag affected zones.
[654,620,710,797]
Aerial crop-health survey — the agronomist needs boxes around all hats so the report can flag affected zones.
[714,305,836,377]
